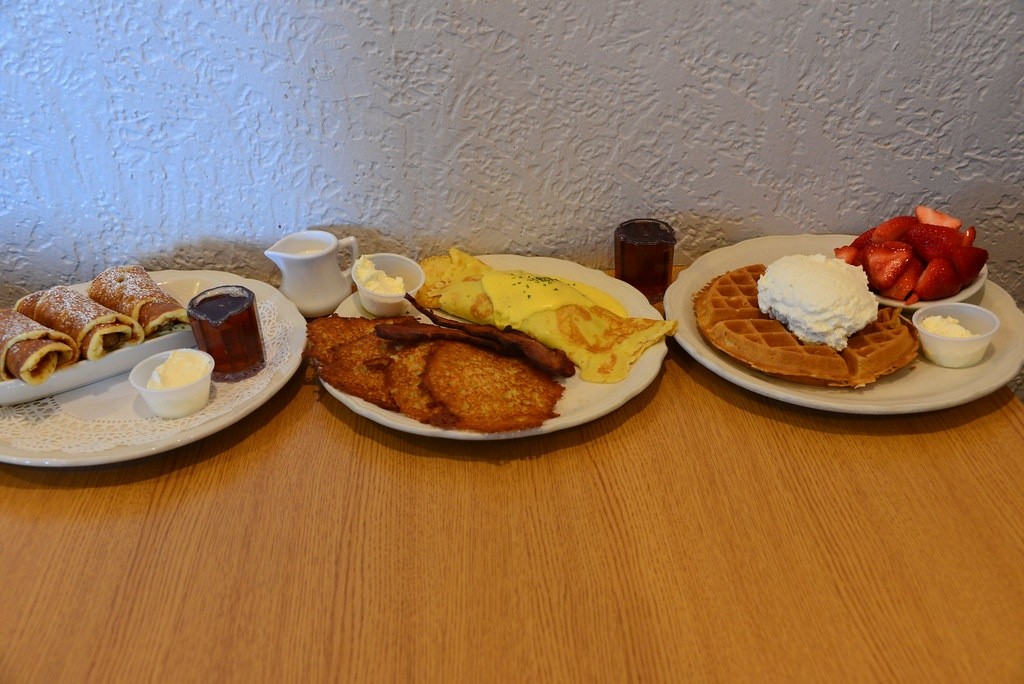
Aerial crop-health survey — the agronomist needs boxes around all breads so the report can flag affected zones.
[695,255,919,387]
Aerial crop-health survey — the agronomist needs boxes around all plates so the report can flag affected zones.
[876,259,987,312]
[314,255,667,439]
[0,276,222,406]
[664,233,1024,412]
[0,270,307,467]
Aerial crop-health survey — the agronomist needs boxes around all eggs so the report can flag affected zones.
[304,294,575,434]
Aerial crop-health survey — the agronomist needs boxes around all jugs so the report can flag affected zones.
[264,230,359,317]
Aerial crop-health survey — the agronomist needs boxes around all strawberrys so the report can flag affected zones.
[833,206,990,306]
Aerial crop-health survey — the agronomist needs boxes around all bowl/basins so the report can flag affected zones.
[351,252,424,317]
[912,302,1000,367]
[128,348,215,418]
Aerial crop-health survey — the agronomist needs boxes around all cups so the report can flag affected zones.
[187,285,267,382]
[614,219,677,304]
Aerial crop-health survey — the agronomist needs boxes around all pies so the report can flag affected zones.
[0,267,192,386]
[414,247,679,384]
